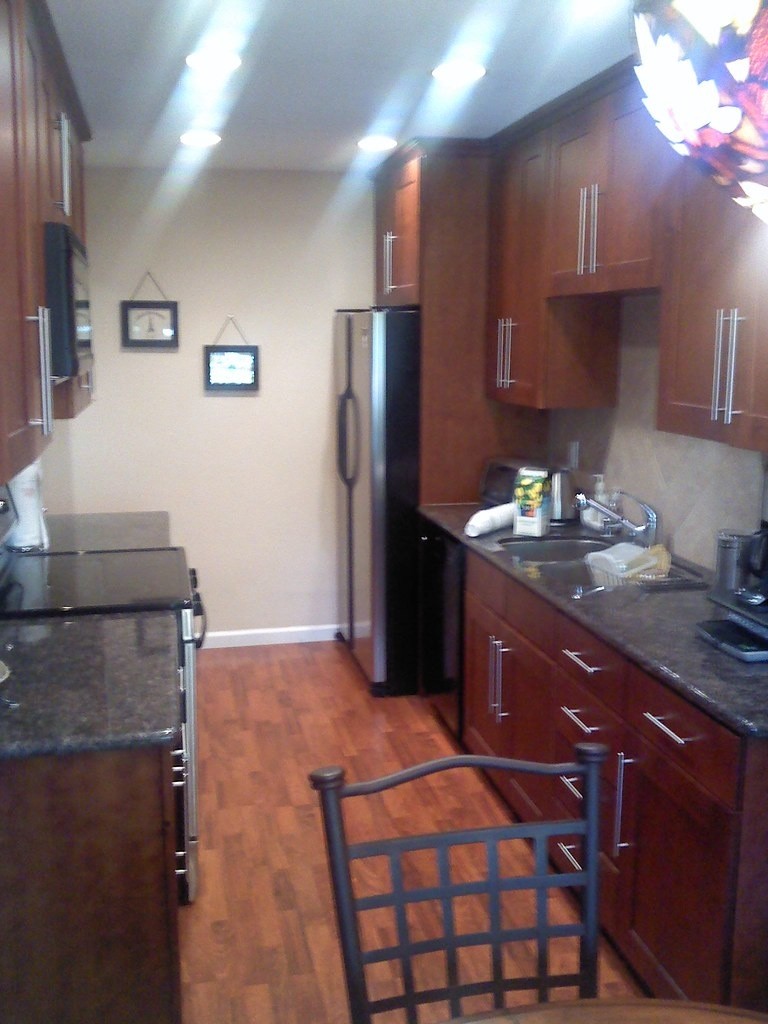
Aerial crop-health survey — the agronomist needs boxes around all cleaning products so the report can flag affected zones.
[575,472,623,532]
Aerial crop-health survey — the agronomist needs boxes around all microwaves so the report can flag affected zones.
[45,220,94,382]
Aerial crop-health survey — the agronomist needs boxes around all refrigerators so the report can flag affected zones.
[331,308,419,697]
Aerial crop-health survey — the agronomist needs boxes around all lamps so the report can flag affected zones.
[633,0,767,224]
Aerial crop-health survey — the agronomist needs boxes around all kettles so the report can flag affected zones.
[545,467,579,526]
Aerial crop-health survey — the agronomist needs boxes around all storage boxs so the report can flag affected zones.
[514,466,550,537]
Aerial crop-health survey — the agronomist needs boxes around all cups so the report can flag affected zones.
[717,529,753,591]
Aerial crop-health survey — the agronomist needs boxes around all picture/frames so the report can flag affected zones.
[205,345,259,391]
[120,300,178,348]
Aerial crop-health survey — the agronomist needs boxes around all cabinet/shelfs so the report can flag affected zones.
[1,609,197,1024]
[0,0,91,484]
[463,549,767,1011]
[656,157,767,452]
[544,51,661,297]
[370,138,488,307]
[487,103,623,410]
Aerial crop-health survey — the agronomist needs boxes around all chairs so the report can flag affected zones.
[308,743,614,1023]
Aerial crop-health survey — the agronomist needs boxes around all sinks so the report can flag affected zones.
[536,560,688,587]
[498,535,616,563]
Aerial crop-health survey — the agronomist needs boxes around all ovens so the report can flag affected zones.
[182,567,208,904]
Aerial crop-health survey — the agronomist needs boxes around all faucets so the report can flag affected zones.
[573,492,659,547]
